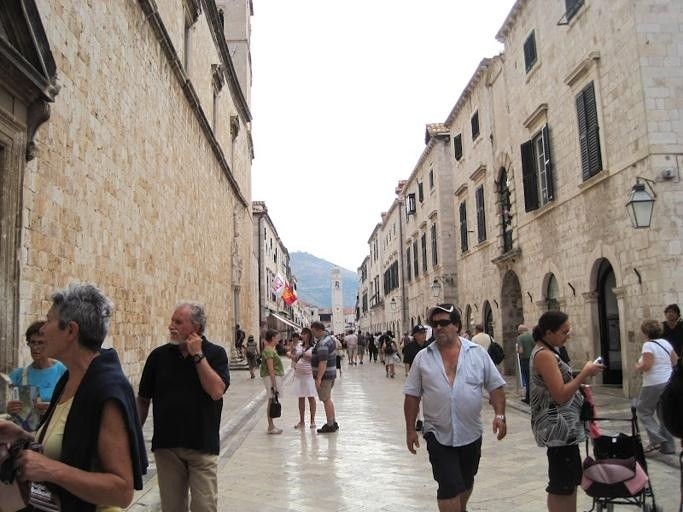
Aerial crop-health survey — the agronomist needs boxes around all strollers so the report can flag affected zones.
[581,405,663,511]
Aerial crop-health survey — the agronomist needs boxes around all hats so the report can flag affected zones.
[413,323,428,331]
[426,304,455,319]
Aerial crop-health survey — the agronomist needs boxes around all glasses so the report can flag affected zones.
[430,320,453,328]
[0,438,31,485]
[27,343,46,347]
[555,325,573,338]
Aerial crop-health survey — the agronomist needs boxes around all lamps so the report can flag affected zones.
[430,276,452,297]
[390,296,401,311]
[624,169,679,229]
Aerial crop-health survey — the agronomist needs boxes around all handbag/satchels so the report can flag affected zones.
[270,403,281,418]
[488,342,505,365]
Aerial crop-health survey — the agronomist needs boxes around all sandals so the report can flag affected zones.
[294,423,305,428]
[267,427,282,434]
[310,421,316,428]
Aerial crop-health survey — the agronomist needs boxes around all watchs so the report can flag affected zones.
[192,353,205,363]
[495,414,506,422]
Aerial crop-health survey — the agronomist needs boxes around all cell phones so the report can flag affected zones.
[594,356,605,365]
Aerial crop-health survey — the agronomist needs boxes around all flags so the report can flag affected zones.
[271,272,285,292]
[281,282,297,306]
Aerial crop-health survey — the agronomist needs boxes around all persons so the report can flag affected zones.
[7,321,68,431]
[529,311,605,512]
[235,321,435,434]
[463,324,535,405]
[0,278,149,512]
[635,319,678,454]
[136,301,230,512]
[403,304,507,512]
[658,304,683,440]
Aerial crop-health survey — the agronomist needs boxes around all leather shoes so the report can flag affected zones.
[416,420,422,431]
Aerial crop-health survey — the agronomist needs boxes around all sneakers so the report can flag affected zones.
[643,441,662,453]
[521,398,529,402]
[317,422,338,433]
